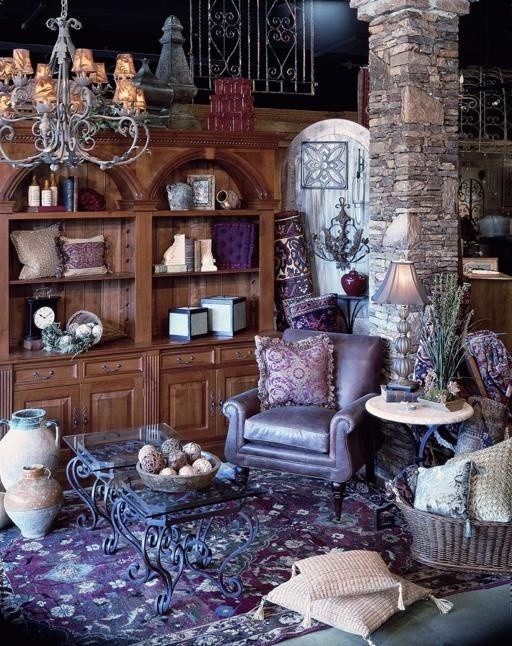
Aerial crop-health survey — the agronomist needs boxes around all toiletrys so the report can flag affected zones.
[28,176,40,207]
[41,179,52,207]
[50,174,58,207]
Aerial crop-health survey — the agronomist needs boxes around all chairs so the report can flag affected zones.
[223,327,386,521]
[462,328,512,440]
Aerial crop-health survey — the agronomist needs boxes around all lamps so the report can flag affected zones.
[0,0,155,174]
[370,259,433,391]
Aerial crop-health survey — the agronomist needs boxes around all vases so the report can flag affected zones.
[0,463,65,542]
[216,189,242,209]
[416,395,467,412]
[0,408,64,489]
[341,270,367,296]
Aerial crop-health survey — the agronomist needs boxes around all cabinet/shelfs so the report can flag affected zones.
[1,347,151,440]
[0,123,143,356]
[150,332,266,449]
[462,267,512,364]
[143,127,279,340]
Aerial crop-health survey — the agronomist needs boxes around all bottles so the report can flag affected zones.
[26,172,58,206]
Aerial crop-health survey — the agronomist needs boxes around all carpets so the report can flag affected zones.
[274,209,315,332]
[0,471,512,645]
[286,292,340,333]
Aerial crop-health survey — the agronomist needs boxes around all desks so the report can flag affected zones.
[363,393,475,530]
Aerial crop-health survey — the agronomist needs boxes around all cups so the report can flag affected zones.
[64,176,79,212]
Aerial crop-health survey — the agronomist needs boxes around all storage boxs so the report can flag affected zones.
[199,293,247,338]
[168,305,209,342]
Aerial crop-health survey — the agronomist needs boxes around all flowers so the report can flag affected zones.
[414,268,479,403]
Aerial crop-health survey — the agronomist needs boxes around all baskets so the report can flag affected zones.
[389,463,512,583]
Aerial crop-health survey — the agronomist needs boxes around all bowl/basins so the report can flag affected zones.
[134,450,221,491]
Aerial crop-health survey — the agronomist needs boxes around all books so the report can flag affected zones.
[63,179,73,212]
[379,384,425,402]
[26,206,66,213]
[69,175,79,211]
[185,239,195,271]
[194,241,201,272]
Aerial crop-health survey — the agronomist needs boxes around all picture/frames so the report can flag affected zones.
[184,173,217,212]
[461,257,499,276]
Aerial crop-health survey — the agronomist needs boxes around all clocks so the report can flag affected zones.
[19,293,62,350]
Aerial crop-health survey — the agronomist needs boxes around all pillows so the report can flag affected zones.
[291,548,410,630]
[246,331,339,413]
[54,232,115,277]
[449,437,512,521]
[413,455,487,538]
[8,219,67,280]
[253,570,456,644]
[209,220,257,271]
